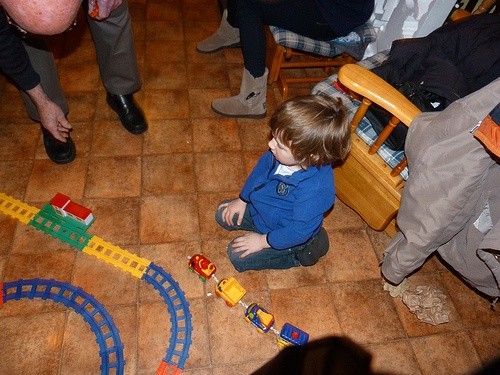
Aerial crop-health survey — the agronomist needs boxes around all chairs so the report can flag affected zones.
[266,0,500,240]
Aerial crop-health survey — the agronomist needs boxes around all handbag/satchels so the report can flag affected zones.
[365,82,445,151]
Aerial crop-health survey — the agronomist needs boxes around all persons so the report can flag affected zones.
[196,0,375,120]
[215,95,351,272]
[0,0,149,165]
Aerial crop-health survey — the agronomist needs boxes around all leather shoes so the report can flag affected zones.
[106,91,148,135]
[40,121,76,163]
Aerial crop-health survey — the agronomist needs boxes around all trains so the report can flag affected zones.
[187,254,310,350]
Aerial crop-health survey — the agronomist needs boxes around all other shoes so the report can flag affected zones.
[295,229,329,265]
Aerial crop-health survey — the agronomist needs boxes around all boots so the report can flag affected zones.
[196,9,240,54]
[211,67,269,119]
